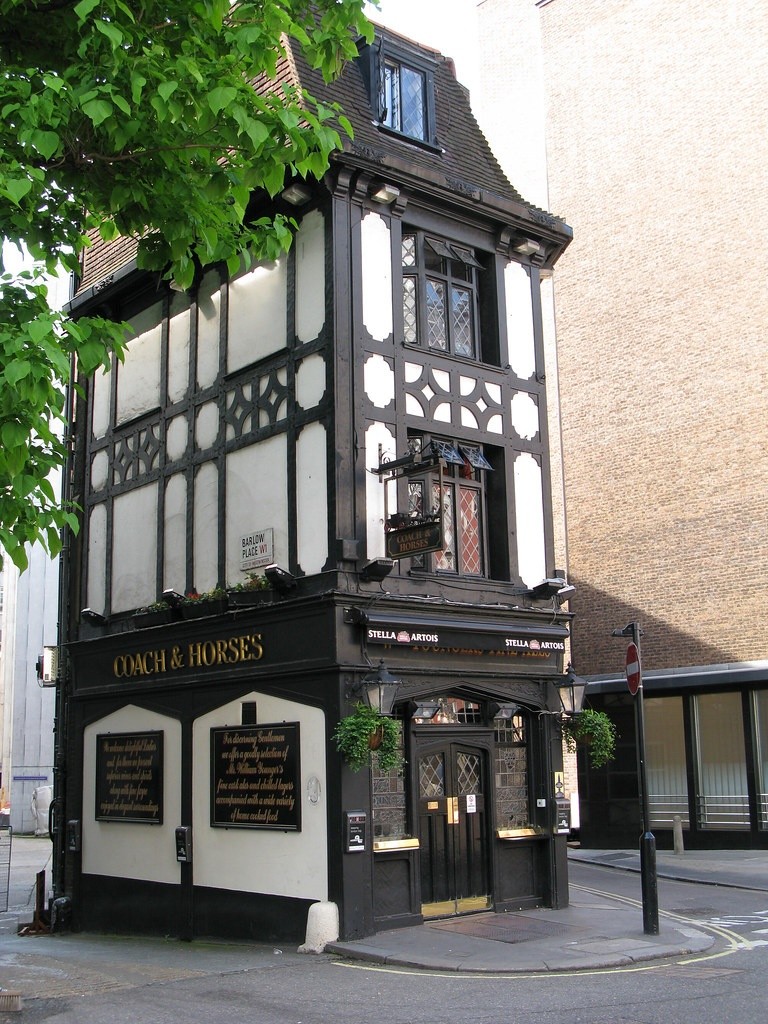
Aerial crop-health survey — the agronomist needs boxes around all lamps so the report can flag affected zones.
[353,658,402,716]
[81,608,106,625]
[552,661,589,732]
[367,183,400,205]
[265,564,296,591]
[161,589,187,609]
[407,701,441,719]
[511,236,539,256]
[362,557,394,581]
[489,702,518,720]
[533,579,578,605]
[282,183,312,206]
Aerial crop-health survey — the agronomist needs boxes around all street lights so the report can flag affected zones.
[610,623,660,938]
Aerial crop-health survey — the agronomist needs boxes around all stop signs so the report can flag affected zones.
[625,641,642,697]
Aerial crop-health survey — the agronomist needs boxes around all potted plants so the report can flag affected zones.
[331,698,409,781]
[130,567,274,629]
[562,708,617,769]
